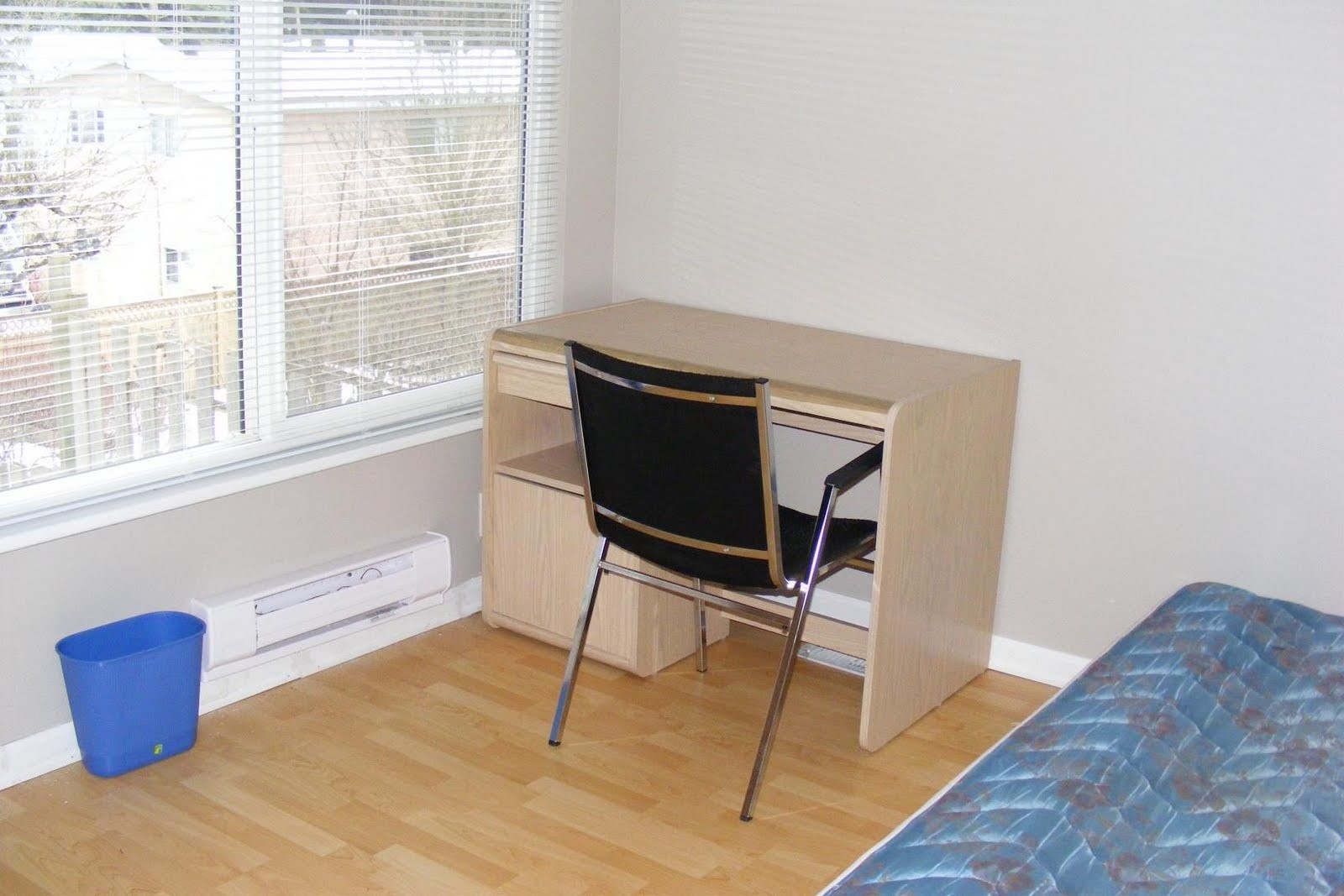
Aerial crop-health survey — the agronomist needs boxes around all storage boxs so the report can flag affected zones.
[56,608,205,779]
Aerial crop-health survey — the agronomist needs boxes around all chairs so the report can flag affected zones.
[547,336,884,820]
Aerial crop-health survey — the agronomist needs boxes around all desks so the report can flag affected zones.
[480,298,1020,752]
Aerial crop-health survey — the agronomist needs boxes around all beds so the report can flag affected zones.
[820,582,1344,896]
